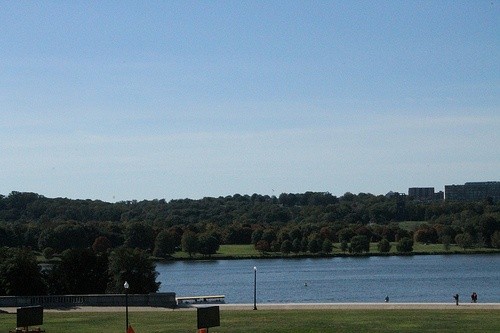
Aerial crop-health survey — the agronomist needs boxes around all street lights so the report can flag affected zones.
[124,281,129,333]
[252,266,257,310]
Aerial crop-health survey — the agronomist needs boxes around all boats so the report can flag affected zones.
[175,295,226,305]
